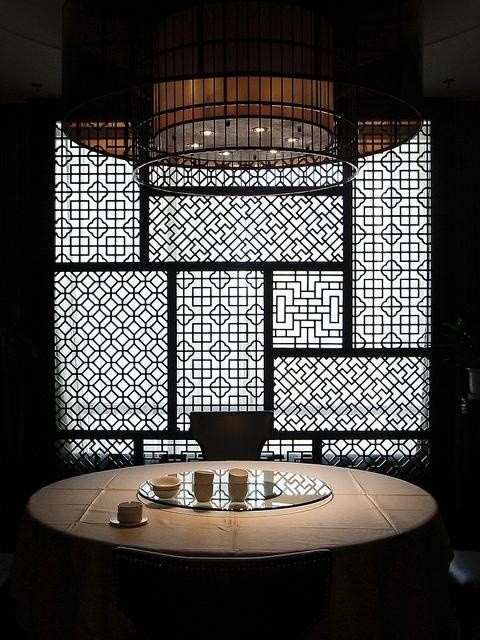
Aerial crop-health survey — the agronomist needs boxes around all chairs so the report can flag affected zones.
[190,408,274,462]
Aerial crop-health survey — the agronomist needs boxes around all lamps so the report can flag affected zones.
[60,0,428,202]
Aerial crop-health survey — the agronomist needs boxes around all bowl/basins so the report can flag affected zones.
[148,477,182,498]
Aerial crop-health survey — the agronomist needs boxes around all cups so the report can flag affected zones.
[192,468,214,501]
[119,501,144,525]
[227,468,249,502]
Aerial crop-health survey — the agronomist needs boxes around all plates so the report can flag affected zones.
[109,515,148,526]
